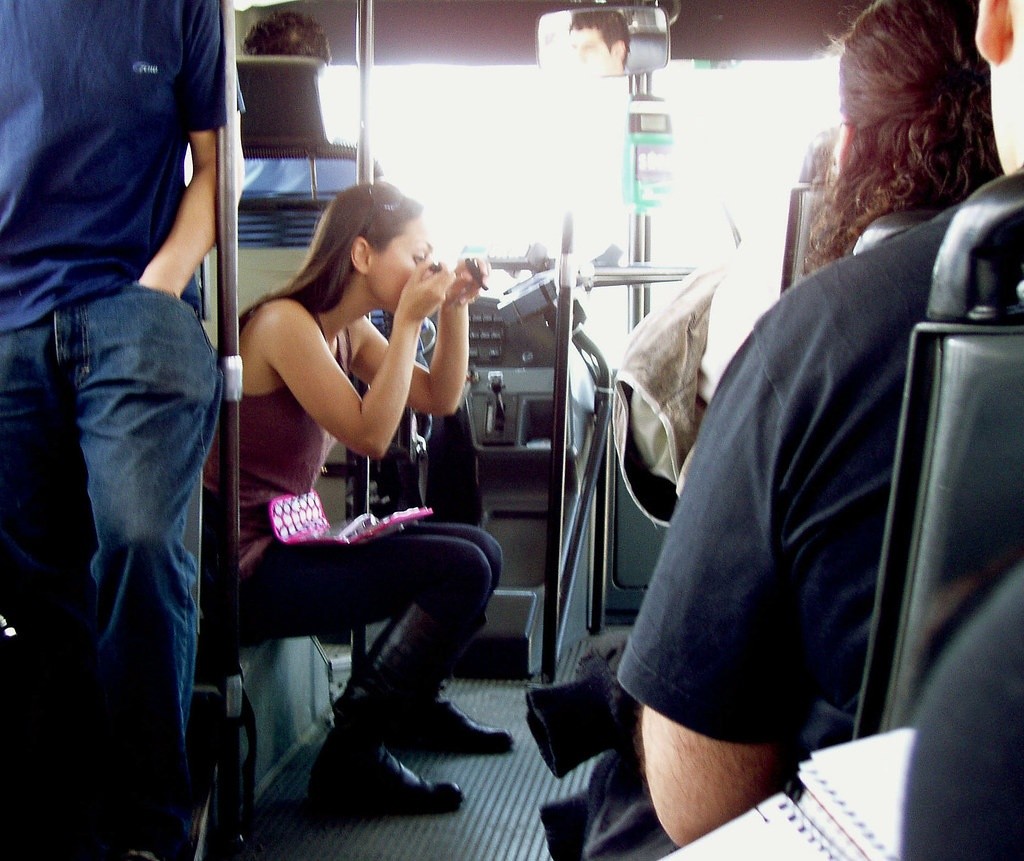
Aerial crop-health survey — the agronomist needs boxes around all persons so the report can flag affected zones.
[238,5,332,58]
[202,181,514,821]
[568,10,631,78]
[902,565,1021,851]
[620,1,1023,849]
[794,0,1004,279]
[3,0,245,851]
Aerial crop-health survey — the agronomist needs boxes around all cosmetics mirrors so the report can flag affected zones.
[465,258,491,292]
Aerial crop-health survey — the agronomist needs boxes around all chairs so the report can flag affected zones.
[778,129,843,296]
[199,55,386,475]
[849,170,1024,742]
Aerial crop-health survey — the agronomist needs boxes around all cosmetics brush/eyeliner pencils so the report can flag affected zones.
[428,261,440,270]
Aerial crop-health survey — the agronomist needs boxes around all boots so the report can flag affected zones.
[387,694,513,755]
[304,601,464,816]
